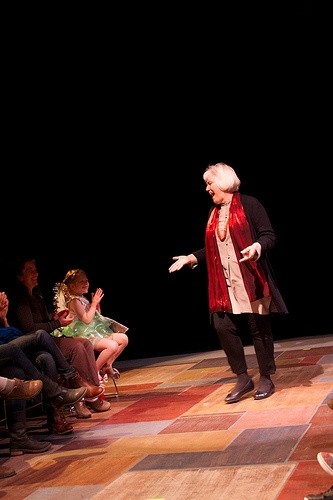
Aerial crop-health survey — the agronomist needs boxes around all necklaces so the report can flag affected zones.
[216,202,232,241]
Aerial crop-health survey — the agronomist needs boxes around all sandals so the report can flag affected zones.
[98,367,120,380]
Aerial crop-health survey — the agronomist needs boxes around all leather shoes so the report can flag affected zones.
[0,465,16,479]
[1,377,42,400]
[224,377,274,402]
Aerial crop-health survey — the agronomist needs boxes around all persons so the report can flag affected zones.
[167,163,290,406]
[0,375,42,478]
[61,268,129,384]
[302,397,333,500]
[6,255,111,419]
[0,289,87,457]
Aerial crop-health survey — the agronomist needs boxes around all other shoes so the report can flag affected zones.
[74,401,92,419]
[88,399,111,412]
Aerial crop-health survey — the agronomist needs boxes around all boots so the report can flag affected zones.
[46,410,74,435]
[4,411,51,456]
[67,374,104,400]
[37,371,87,408]
